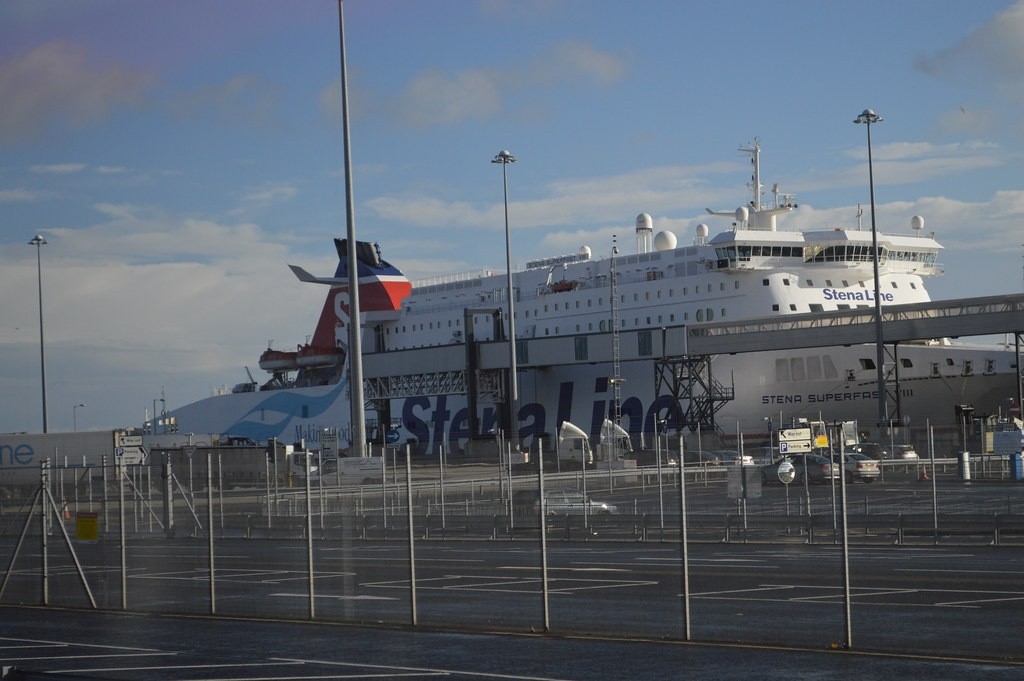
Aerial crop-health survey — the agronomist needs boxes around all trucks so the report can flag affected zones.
[590,419,633,463]
[507,421,593,471]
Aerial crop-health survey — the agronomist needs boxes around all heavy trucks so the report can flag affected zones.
[128,434,259,492]
[149,444,326,491]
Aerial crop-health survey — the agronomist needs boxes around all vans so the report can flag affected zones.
[321,457,382,485]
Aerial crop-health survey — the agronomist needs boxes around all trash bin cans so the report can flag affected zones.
[1010,453,1021,481]
[727,466,763,500]
[958,451,971,480]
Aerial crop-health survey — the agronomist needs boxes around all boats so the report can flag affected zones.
[133,136,1024,460]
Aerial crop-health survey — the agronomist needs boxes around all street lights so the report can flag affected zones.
[27,233,49,434]
[154,399,164,434]
[493,148,527,462]
[74,404,84,431]
[855,110,889,439]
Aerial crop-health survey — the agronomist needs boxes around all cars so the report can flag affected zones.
[826,451,881,482]
[620,449,743,481]
[844,443,920,472]
[509,490,619,524]
[750,454,840,498]
